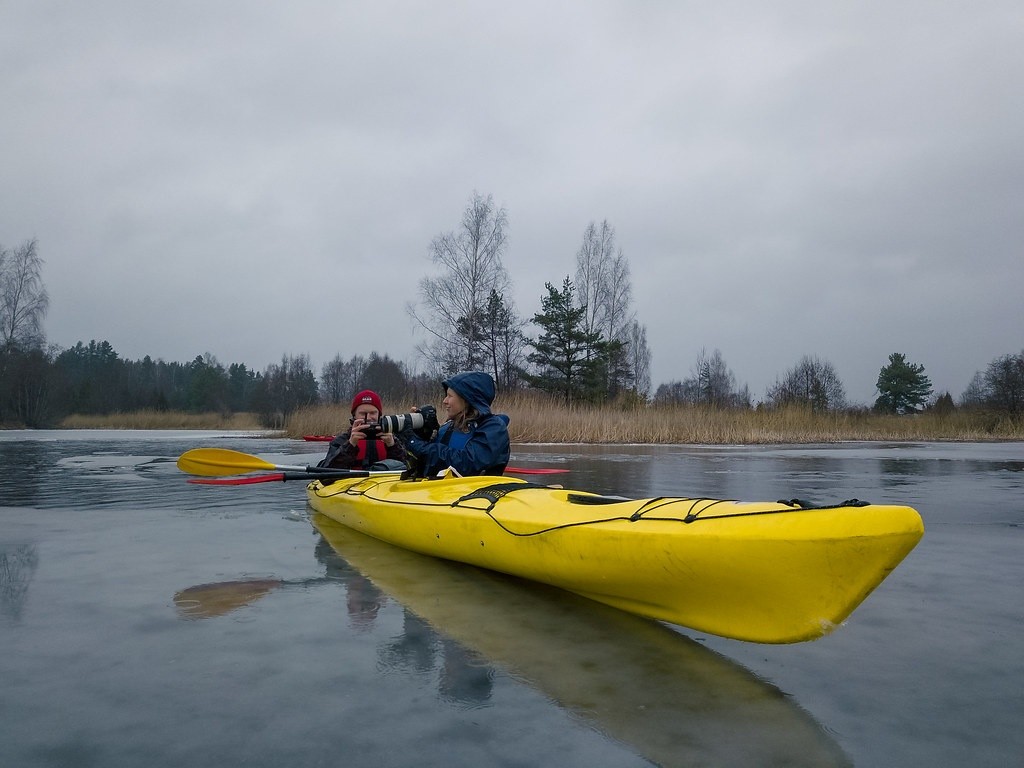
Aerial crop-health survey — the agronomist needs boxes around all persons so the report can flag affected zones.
[317,390,407,487]
[396,372,511,482]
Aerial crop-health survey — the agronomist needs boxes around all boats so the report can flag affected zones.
[306,506,851,767]
[305,467,924,645]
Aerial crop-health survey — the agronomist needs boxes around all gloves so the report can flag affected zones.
[418,404,437,422]
[394,418,421,444]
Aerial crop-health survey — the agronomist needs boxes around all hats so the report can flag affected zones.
[351,389,382,415]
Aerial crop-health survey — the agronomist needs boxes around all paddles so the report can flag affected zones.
[176,448,574,489]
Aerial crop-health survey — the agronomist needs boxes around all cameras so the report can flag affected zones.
[380,404,439,435]
[358,422,381,440]
